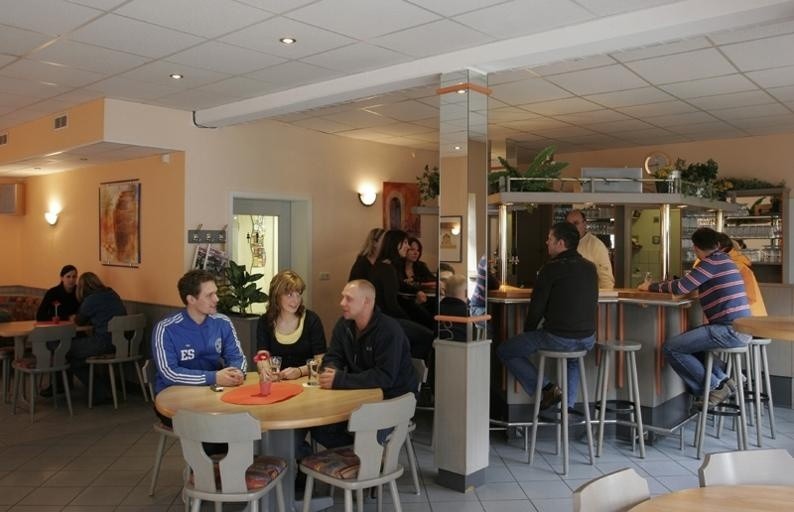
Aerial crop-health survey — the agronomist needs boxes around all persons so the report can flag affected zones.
[310,279,419,501]
[441,274,468,341]
[689,233,771,395]
[438,264,454,299]
[562,207,618,292]
[249,270,331,493]
[147,269,252,456]
[366,229,438,404]
[468,253,487,329]
[68,272,131,407]
[639,230,752,411]
[31,263,85,398]
[348,225,383,279]
[494,222,599,417]
[392,237,434,283]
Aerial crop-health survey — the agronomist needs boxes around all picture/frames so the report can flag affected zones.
[440,215,463,263]
[96,178,142,269]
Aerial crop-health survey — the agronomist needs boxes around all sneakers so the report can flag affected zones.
[726,378,737,393]
[707,381,733,411]
[39,378,73,398]
[89,375,106,407]
[742,373,748,384]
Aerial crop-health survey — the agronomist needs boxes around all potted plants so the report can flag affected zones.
[654,155,718,196]
[217,260,270,371]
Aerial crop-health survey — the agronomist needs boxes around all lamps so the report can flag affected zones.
[45,213,58,226]
[357,193,377,206]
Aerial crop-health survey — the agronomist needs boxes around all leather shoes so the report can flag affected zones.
[539,383,564,412]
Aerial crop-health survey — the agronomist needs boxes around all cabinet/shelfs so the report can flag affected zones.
[679,187,794,284]
[512,199,630,288]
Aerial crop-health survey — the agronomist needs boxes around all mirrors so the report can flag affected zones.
[439,71,490,343]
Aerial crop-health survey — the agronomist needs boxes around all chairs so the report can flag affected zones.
[83,312,150,413]
[142,359,181,498]
[369,357,431,499]
[572,466,650,512]
[698,446,793,488]
[298,392,418,512]
[12,322,75,424]
[170,408,289,511]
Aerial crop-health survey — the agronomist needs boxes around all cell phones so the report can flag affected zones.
[211,384,224,392]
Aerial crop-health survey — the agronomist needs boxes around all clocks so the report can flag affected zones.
[644,151,671,177]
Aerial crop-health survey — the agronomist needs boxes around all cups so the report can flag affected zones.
[269,357,282,381]
[686,212,780,265]
[306,360,318,384]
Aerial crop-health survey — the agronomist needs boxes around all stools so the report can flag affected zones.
[1,344,20,403]
[692,341,751,459]
[525,351,596,476]
[715,332,777,448]
[594,337,648,459]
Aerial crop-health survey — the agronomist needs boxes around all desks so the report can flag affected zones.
[733,314,794,343]
